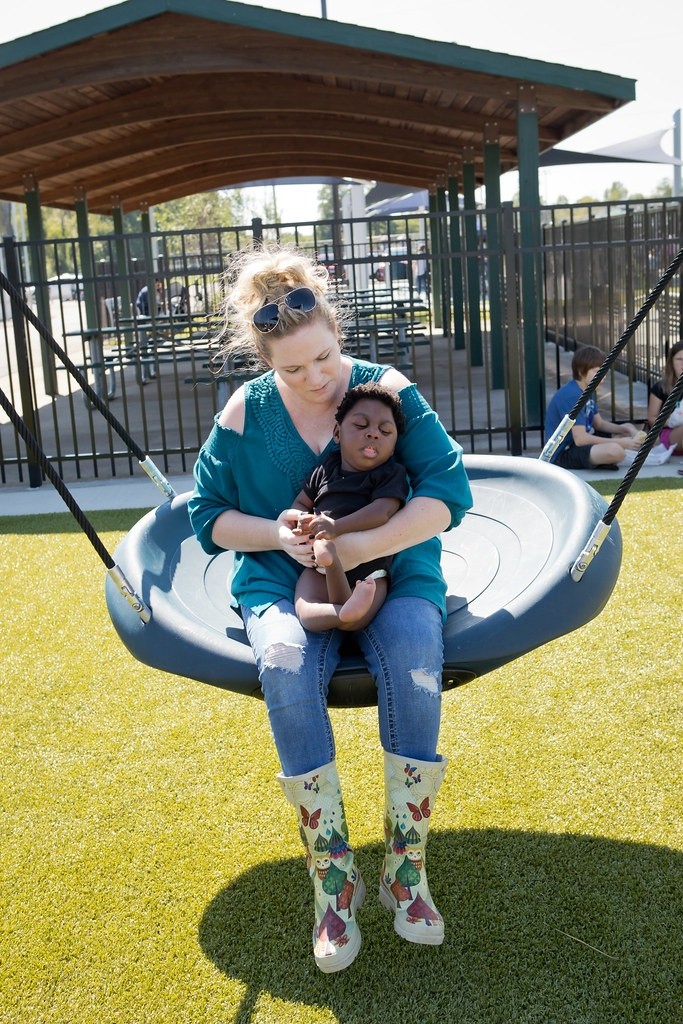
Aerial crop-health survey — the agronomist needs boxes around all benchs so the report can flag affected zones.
[55,285,432,416]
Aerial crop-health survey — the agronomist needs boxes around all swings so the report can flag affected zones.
[0,245,681,711]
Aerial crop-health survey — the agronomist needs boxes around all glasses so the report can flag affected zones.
[254,287,316,333]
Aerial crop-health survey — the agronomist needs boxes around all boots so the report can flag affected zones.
[379,750,449,944]
[275,761,367,973]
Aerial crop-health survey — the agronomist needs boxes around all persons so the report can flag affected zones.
[544,344,645,470]
[648,341,683,457]
[413,245,431,303]
[290,381,417,637]
[135,277,166,324]
[185,241,474,976]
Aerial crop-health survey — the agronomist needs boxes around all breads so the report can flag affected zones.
[632,431,647,442]
